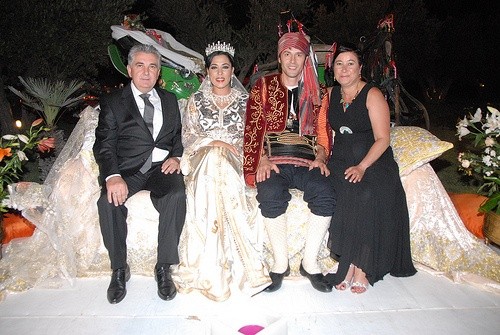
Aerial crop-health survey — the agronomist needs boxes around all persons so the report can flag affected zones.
[181,41,273,302]
[324,41,417,294]
[244,20,333,293]
[93,45,186,304]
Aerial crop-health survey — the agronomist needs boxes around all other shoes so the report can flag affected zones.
[299,260,332,293]
[264,262,290,292]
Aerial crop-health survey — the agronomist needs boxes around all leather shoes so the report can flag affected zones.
[154,264,177,301]
[106,264,131,304]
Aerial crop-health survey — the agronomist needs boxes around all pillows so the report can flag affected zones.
[448,190,489,239]
[390,127,453,176]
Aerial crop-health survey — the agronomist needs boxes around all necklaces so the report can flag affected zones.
[339,85,360,111]
[210,90,235,104]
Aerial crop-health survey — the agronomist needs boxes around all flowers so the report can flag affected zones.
[456,105,500,213]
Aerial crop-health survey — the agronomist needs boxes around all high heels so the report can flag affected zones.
[336,263,369,295]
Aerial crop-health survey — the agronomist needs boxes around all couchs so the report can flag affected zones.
[0,103,500,302]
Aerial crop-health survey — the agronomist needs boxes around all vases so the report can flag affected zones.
[482,208,500,246]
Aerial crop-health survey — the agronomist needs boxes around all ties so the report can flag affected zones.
[138,92,155,175]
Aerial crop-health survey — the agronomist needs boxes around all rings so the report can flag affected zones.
[111,191,117,195]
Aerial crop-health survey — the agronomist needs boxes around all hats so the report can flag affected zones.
[278,33,323,109]
